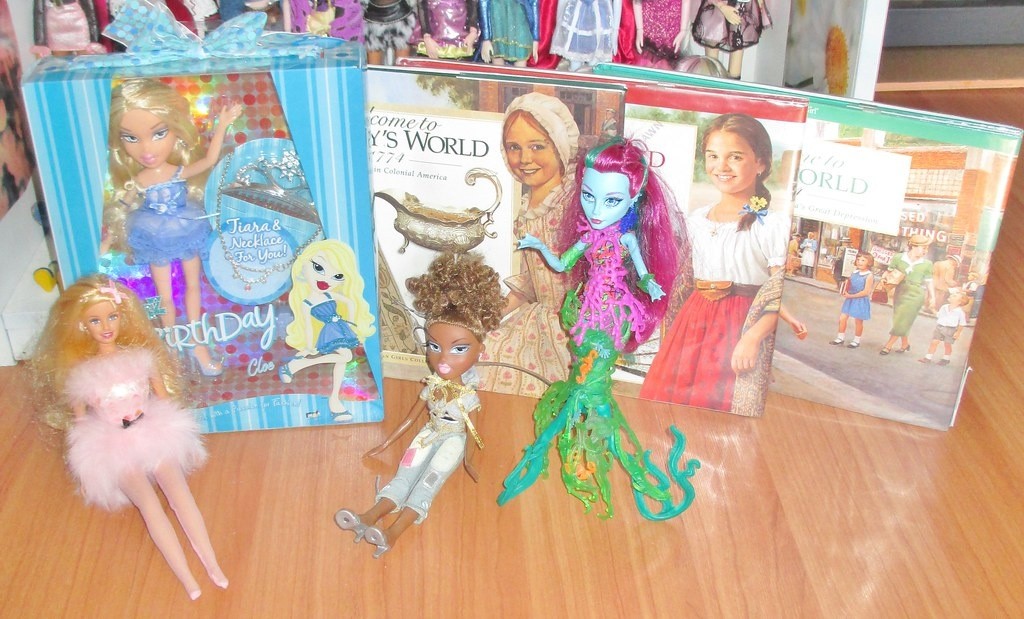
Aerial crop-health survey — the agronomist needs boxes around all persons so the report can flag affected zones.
[334,249,509,558]
[31,0,97,59]
[218,0,690,71]
[101,78,243,376]
[691,0,773,80]
[29,274,229,600]
[497,136,701,520]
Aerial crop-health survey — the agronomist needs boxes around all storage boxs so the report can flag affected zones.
[19,43,384,437]
